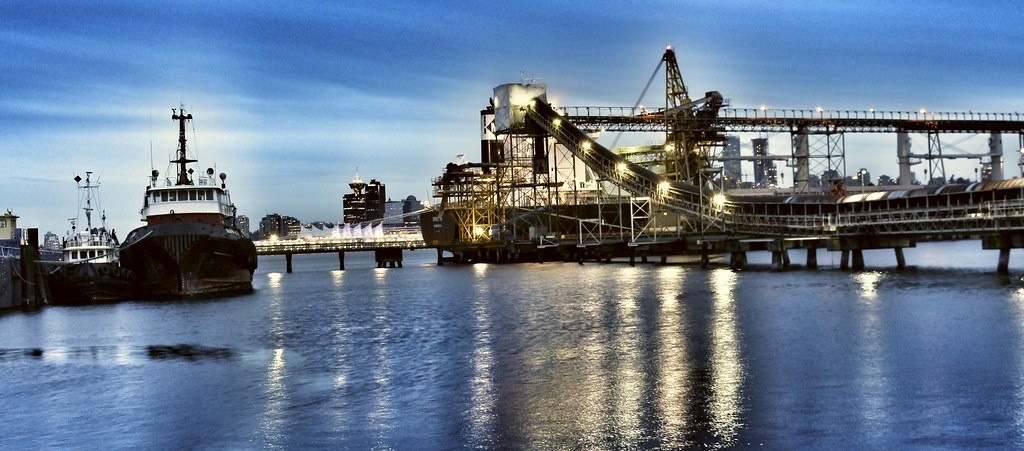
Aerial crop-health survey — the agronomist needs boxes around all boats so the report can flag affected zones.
[46,171,140,304]
[119,102,258,302]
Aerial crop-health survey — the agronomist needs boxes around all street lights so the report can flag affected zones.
[858,169,867,193]
